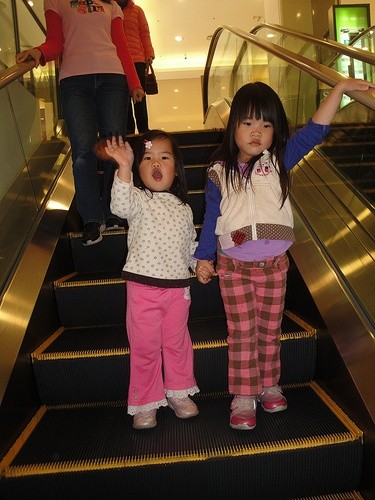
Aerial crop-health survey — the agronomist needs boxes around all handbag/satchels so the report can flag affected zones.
[145,62,158,95]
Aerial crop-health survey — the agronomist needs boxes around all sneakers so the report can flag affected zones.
[133,410,159,428]
[105,218,126,231]
[167,396,201,418]
[230,393,257,430]
[82,221,106,246]
[257,384,288,413]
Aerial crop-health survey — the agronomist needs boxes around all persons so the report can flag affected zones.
[18,0,144,247]
[195,78,375,430]
[116,1,155,134]
[97,129,216,430]
